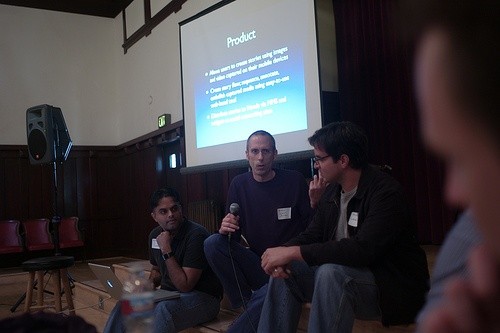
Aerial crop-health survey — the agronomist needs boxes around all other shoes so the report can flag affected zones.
[240,287,259,309]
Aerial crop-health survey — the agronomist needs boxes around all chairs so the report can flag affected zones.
[0,216,85,261]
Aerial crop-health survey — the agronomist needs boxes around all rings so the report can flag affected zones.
[273,268,277,272]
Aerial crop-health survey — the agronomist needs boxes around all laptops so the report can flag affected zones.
[88,263,181,305]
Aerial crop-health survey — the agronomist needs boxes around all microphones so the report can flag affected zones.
[228,203,240,238]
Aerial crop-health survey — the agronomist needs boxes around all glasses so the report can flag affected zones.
[312,154,331,163]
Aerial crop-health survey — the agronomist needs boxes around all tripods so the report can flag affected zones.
[10,163,77,312]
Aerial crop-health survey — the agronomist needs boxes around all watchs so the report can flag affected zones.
[162,251,174,261]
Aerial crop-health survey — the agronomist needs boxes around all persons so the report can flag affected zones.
[257,121,430,333]
[204,130,330,333]
[414,0,500,333]
[103,189,224,333]
[0,311,97,333]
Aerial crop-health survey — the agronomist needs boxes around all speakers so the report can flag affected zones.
[26,104,72,166]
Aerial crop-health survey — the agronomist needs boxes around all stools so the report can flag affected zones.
[21,255,75,317]
